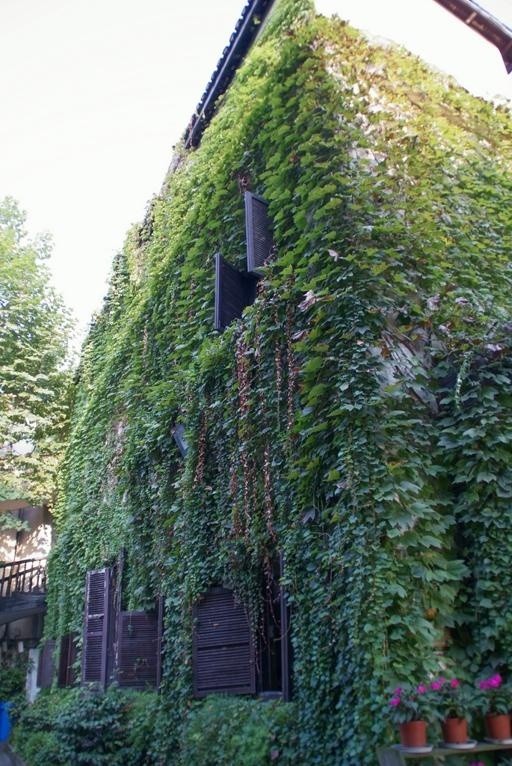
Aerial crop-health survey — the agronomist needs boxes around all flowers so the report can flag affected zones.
[384,674,511,725]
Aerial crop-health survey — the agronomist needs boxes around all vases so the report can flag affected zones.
[400,714,511,748]
